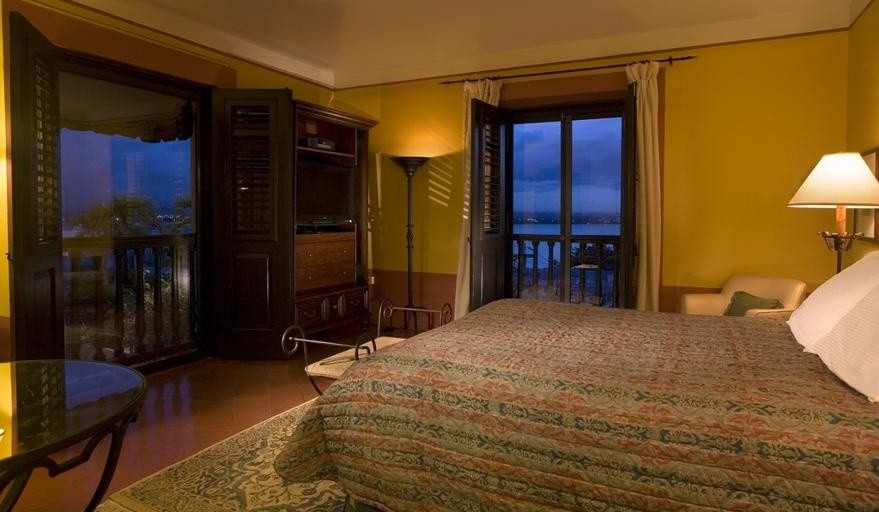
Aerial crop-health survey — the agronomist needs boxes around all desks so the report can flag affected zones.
[0,356,150,512]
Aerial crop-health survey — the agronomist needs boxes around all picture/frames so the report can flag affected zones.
[847,147,879,243]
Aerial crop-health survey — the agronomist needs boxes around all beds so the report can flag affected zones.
[263,241,879,509]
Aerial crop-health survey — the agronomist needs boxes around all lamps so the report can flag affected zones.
[385,153,432,344]
[784,147,879,277]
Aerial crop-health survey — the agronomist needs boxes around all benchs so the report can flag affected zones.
[274,294,457,404]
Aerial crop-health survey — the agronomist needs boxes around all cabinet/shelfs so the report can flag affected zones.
[295,99,379,364]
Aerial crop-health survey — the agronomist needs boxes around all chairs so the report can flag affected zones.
[673,268,808,320]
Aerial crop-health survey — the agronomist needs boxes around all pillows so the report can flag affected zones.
[797,281,879,407]
[720,289,784,320]
[780,247,879,356]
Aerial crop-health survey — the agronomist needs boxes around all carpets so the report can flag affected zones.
[91,390,383,512]
